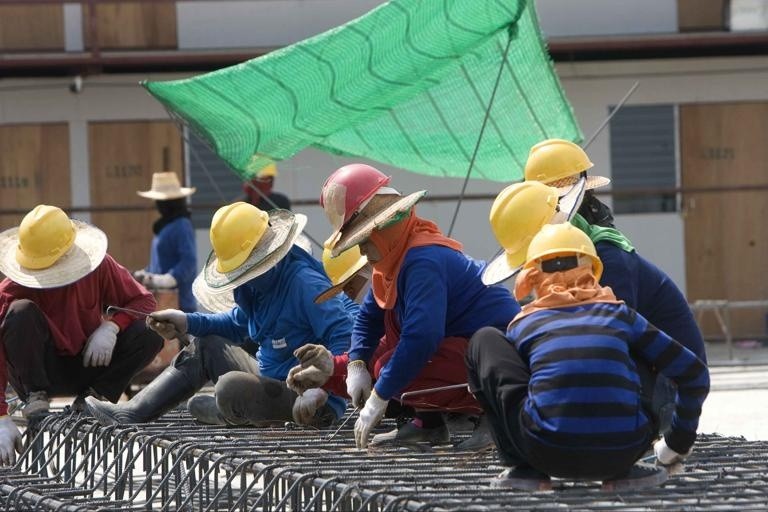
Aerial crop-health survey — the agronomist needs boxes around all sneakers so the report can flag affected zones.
[600,462,669,496]
[491,463,542,496]
[23,389,49,418]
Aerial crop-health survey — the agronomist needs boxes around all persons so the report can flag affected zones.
[235,157,291,211]
[287,234,369,397]
[481,181,706,368]
[321,164,519,450]
[85,202,356,429]
[465,221,709,492]
[135,171,199,313]
[525,138,616,230]
[0,205,164,465]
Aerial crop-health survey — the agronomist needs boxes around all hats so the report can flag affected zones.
[136,171,197,199]
[313,279,355,311]
[328,186,428,260]
[480,176,586,287]
[192,207,308,297]
[191,268,238,313]
[0,216,107,290]
[546,174,611,190]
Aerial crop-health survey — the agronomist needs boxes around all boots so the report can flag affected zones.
[186,392,231,427]
[84,365,197,427]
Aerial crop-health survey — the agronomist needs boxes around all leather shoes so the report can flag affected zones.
[371,417,452,450]
[456,411,496,452]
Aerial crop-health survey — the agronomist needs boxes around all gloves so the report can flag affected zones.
[82,318,119,370]
[293,385,328,424]
[345,359,373,406]
[652,436,694,464]
[0,416,26,465]
[284,364,323,391]
[292,342,336,377]
[353,389,389,452]
[148,308,189,340]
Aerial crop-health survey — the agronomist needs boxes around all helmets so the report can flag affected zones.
[489,182,561,269]
[523,221,604,280]
[524,138,595,184]
[320,235,369,285]
[319,164,392,224]
[15,202,77,270]
[252,152,278,181]
[208,201,270,275]
